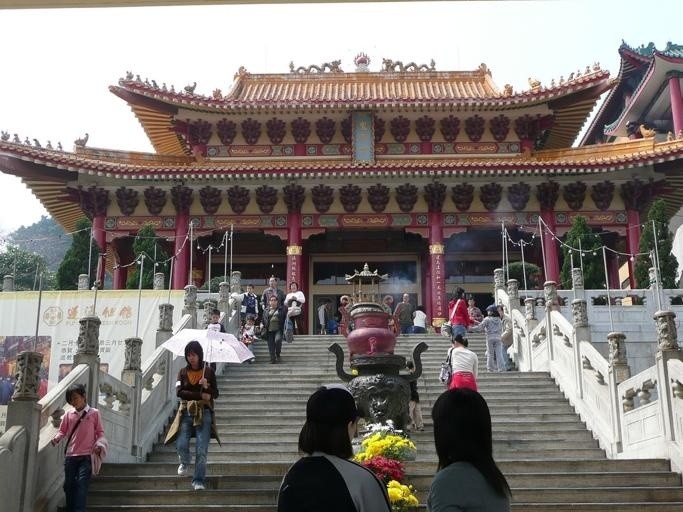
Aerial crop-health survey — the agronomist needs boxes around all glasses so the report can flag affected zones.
[270,299,277,301]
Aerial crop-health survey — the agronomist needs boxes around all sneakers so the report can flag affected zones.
[415,425,424,431]
[250,358,256,363]
[177,463,191,476]
[192,479,205,491]
[269,359,274,364]
[277,357,281,361]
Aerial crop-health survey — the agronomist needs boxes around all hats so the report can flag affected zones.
[486,305,497,311]
[307,387,357,423]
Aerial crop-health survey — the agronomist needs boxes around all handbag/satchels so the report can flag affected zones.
[288,307,302,317]
[259,328,268,340]
[441,321,452,339]
[439,348,454,385]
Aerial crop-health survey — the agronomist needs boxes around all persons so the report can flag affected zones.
[338,293,351,336]
[392,292,414,333]
[230,283,258,322]
[405,359,425,433]
[441,332,479,393]
[260,276,287,338]
[467,305,506,373]
[237,314,262,364]
[384,295,395,332]
[276,385,392,511]
[484,304,514,372]
[467,297,483,327]
[202,309,225,375]
[0,375,47,405]
[51,384,106,512]
[327,314,342,336]
[165,340,221,491]
[450,284,480,347]
[413,304,427,334]
[259,295,286,363]
[283,280,305,335]
[317,300,332,335]
[427,386,514,511]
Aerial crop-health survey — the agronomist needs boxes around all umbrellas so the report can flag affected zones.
[161,328,255,383]
